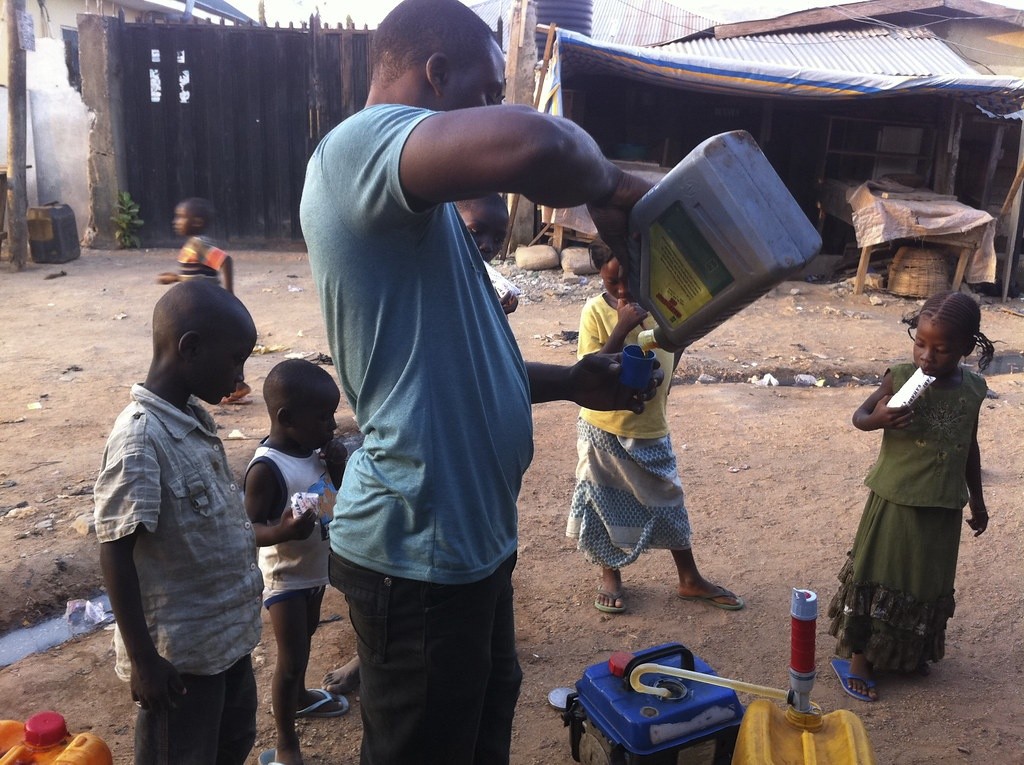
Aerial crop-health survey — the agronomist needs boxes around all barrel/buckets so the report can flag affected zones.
[26,201,81,264]
[606,130,823,352]
[562,642,747,765]
[0,712,112,765]
[732,699,874,765]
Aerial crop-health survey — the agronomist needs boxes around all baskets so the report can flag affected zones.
[882,243,950,298]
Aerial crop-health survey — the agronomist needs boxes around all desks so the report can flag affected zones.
[812,178,989,298]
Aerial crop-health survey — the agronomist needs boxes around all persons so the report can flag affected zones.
[826,291,993,702]
[92,277,264,765]
[160,198,253,406]
[243,357,347,765]
[320,192,520,693]
[299,0,665,765]
[565,233,744,613]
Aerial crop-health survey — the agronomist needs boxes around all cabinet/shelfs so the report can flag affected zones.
[812,111,937,188]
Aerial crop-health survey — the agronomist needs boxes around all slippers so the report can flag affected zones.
[830,657,879,702]
[258,749,286,765]
[916,661,931,676]
[593,581,626,612]
[269,688,349,717]
[677,581,743,610]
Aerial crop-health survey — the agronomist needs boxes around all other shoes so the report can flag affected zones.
[221,380,252,405]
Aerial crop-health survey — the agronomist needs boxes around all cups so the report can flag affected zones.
[620,345,656,389]
